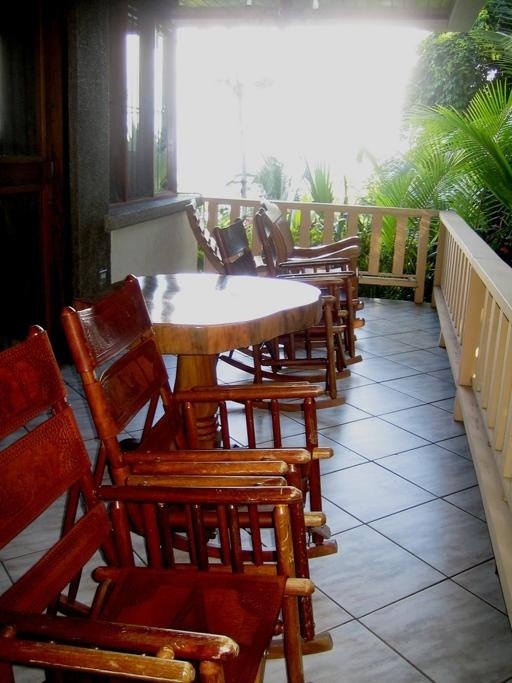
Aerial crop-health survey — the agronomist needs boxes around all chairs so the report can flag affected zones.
[183,199,368,412]
